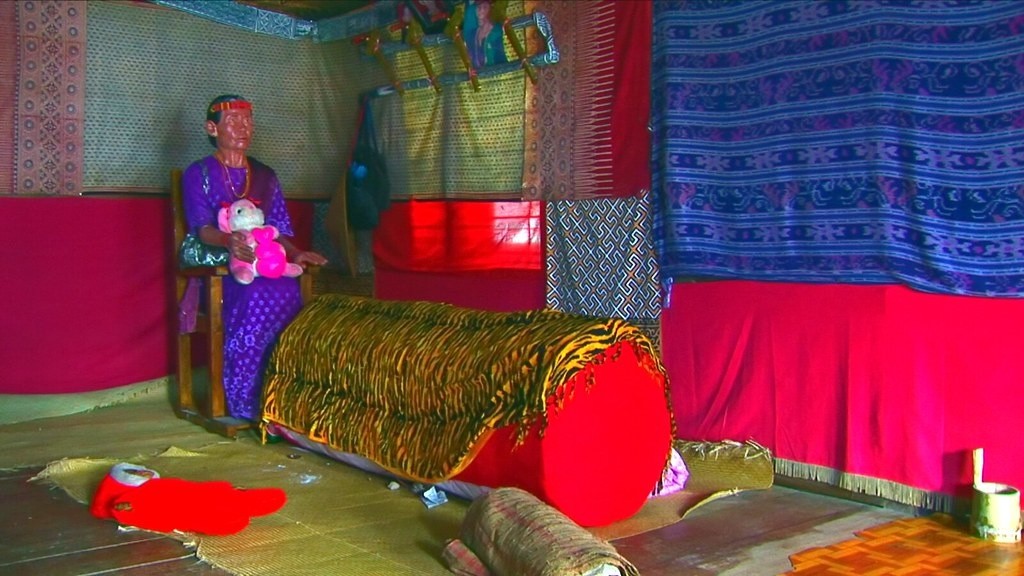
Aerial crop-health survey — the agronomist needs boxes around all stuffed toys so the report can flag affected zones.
[215,196,304,285]
[89,462,287,538]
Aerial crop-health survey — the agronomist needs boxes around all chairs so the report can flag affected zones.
[168,167,321,435]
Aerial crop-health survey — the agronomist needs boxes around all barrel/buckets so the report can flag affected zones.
[969,483,1022,543]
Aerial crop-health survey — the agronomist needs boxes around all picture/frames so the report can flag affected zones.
[407,0,455,35]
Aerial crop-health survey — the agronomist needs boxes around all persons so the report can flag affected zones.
[184,95,329,422]
